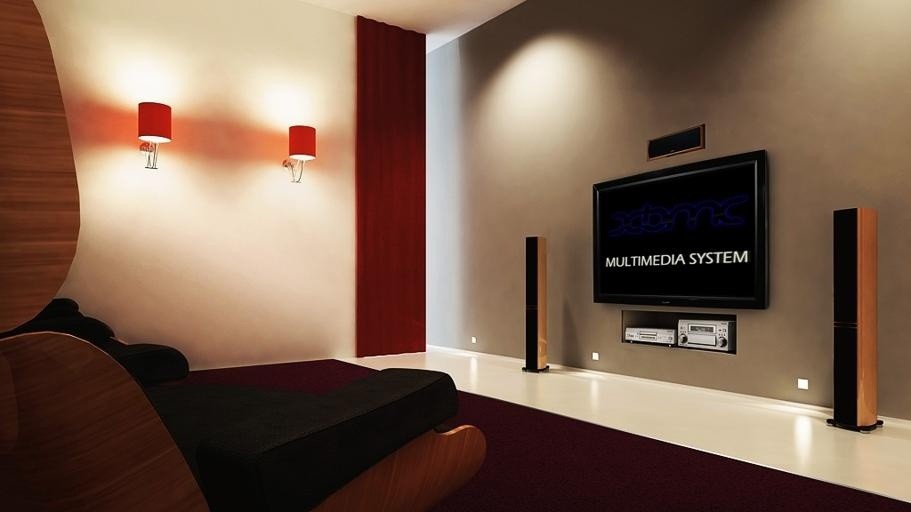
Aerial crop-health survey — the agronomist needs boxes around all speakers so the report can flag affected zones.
[826,207,884,432]
[522,236,549,373]
[648,126,703,159]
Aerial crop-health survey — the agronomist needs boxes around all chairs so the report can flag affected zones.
[1,1,489,512]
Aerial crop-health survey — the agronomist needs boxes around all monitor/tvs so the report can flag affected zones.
[592,149,771,310]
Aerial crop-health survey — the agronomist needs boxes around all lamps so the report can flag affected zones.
[281,125,316,184]
[137,101,173,170]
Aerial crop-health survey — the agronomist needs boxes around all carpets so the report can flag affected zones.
[158,360,909,512]
